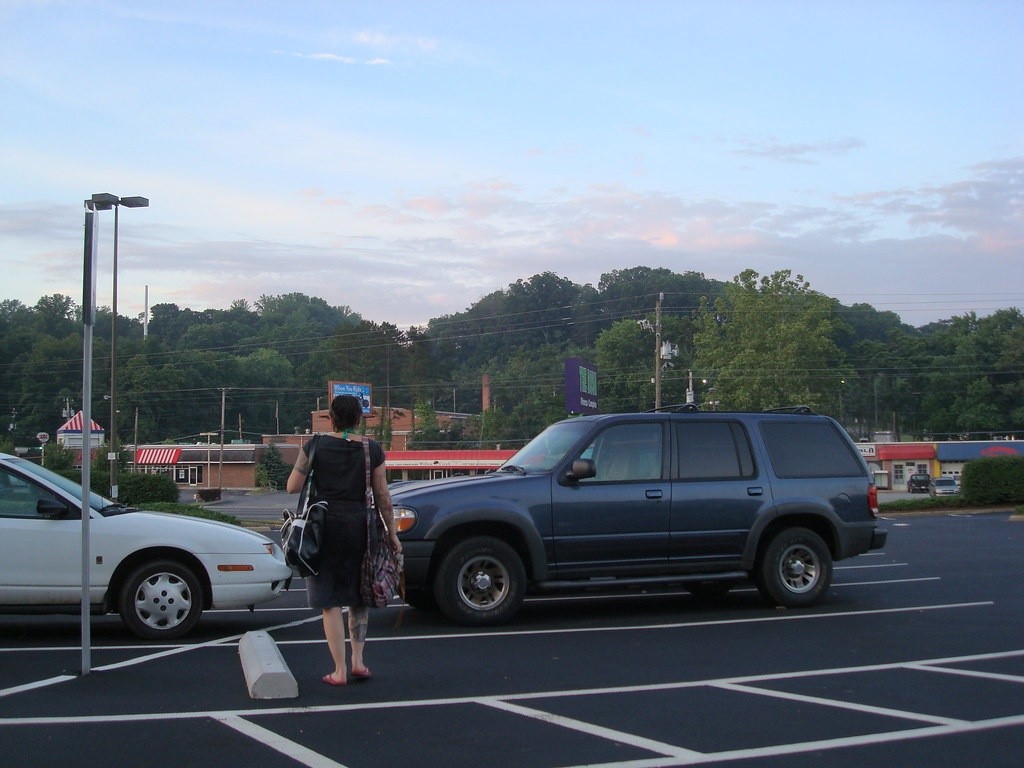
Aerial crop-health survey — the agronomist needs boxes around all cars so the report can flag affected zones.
[907,473,931,493]
[927,478,960,497]
[0,452,292,640]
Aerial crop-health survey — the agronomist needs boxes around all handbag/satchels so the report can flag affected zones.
[360,543,404,608]
[279,494,328,577]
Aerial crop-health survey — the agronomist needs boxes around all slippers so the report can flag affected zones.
[321,674,347,685]
[351,666,373,678]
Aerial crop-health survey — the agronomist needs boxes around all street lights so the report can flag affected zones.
[79,200,112,672]
[91,192,148,502]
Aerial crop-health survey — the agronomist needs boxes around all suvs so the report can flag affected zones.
[385,399,889,628]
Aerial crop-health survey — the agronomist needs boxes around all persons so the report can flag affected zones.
[286,394,402,687]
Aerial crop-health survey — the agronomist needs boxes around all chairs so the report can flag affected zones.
[605,449,661,480]
